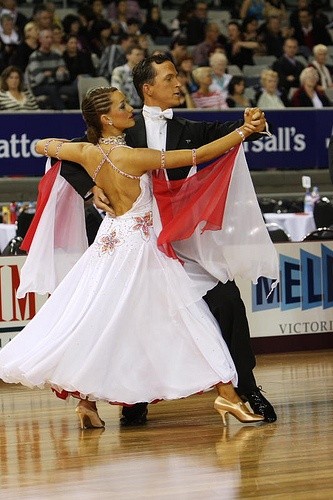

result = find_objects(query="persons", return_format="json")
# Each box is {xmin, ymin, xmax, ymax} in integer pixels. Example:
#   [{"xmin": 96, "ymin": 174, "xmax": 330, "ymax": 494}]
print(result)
[
  {"xmin": 303, "ymin": 44, "xmax": 333, "ymax": 88},
  {"xmin": 289, "ymin": 66, "xmax": 333, "ymax": 108},
  {"xmin": 252, "ymin": 68, "xmax": 289, "ymax": 109},
  {"xmin": 187, "ymin": 67, "xmax": 230, "ymax": 109},
  {"xmin": 0, "ymin": 0, "xmax": 333, "ymax": 78},
  {"xmin": 225, "ymin": 74, "xmax": 256, "ymax": 109},
  {"xmin": 0, "ymin": 65, "xmax": 43, "ymax": 112},
  {"xmin": 33, "ymin": 87, "xmax": 268, "ymax": 430},
  {"xmin": 56, "ymin": 51, "xmax": 278, "ymax": 427},
  {"xmin": 110, "ymin": 46, "xmax": 147, "ymax": 109},
  {"xmin": 167, "ymin": 72, "xmax": 195, "ymax": 109},
  {"xmin": 269, "ymin": 39, "xmax": 306, "ymax": 95},
  {"xmin": 24, "ymin": 29, "xmax": 79, "ymax": 110},
  {"xmin": 195, "ymin": 53, "xmax": 235, "ymax": 96}
]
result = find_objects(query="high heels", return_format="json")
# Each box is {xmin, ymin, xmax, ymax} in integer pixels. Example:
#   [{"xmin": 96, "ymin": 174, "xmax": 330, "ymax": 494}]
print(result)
[
  {"xmin": 78, "ymin": 402, "xmax": 105, "ymax": 429},
  {"xmin": 213, "ymin": 397, "xmax": 264, "ymax": 426}
]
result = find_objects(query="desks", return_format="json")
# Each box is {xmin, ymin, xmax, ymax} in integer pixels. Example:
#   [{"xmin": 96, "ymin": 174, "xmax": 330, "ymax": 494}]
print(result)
[
  {"xmin": 0, "ymin": 223, "xmax": 19, "ymax": 254},
  {"xmin": 263, "ymin": 213, "xmax": 316, "ymax": 242}
]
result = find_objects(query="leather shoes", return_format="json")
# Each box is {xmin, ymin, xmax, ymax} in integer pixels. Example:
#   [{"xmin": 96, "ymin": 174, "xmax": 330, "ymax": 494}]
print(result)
[
  {"xmin": 119, "ymin": 404, "xmax": 149, "ymax": 424},
  {"xmin": 239, "ymin": 385, "xmax": 277, "ymax": 422}
]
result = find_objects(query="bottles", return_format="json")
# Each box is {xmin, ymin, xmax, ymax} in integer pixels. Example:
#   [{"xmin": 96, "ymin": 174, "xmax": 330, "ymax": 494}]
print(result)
[
  {"xmin": 9, "ymin": 199, "xmax": 35, "ymax": 223},
  {"xmin": 304, "ymin": 184, "xmax": 320, "ymax": 213}
]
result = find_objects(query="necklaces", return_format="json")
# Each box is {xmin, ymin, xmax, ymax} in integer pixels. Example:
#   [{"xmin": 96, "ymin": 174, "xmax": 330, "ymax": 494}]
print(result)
[{"xmin": 97, "ymin": 131, "xmax": 129, "ymax": 144}]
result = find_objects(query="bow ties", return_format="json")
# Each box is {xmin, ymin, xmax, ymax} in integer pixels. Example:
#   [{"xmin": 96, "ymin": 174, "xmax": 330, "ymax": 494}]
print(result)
[{"xmin": 142, "ymin": 105, "xmax": 175, "ymax": 119}]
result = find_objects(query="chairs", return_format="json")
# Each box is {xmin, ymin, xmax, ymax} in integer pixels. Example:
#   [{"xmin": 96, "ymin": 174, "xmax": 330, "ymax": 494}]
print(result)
[
  {"xmin": 9, "ymin": 207, "xmax": 36, "ymax": 256},
  {"xmin": 258, "ymin": 197, "xmax": 333, "ymax": 242},
  {"xmin": 13, "ymin": 8, "xmax": 333, "ymax": 109}
]
